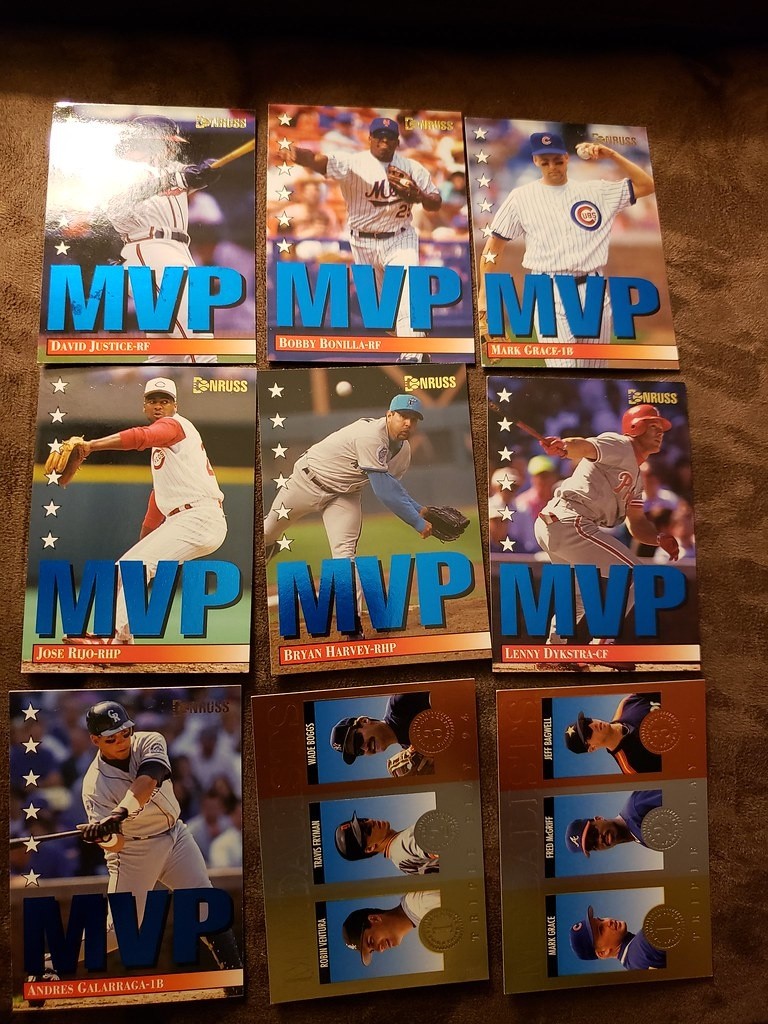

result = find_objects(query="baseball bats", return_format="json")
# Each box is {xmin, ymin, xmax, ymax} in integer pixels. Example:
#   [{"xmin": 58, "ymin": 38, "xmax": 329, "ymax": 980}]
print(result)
[
  {"xmin": 487, "ymin": 397, "xmax": 568, "ymax": 459},
  {"xmin": 9, "ymin": 822, "xmax": 123, "ymax": 851},
  {"xmin": 211, "ymin": 138, "xmax": 256, "ymax": 169}
]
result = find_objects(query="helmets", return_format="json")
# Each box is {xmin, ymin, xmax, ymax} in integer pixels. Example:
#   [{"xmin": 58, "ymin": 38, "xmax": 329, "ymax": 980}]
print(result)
[
  {"xmin": 622, "ymin": 405, "xmax": 672, "ymax": 437},
  {"xmin": 86, "ymin": 702, "xmax": 137, "ymax": 737},
  {"xmin": 127, "ymin": 116, "xmax": 189, "ymax": 144}
]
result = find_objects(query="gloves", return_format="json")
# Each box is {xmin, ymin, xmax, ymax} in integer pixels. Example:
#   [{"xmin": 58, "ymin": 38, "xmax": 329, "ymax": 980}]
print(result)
[
  {"xmin": 75, "ymin": 807, "xmax": 131, "ymax": 842},
  {"xmin": 176, "ymin": 158, "xmax": 223, "ymax": 191}
]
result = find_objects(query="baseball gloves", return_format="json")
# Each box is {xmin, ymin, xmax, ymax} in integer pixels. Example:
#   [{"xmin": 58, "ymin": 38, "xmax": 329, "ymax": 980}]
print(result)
[
  {"xmin": 45, "ymin": 433, "xmax": 87, "ymax": 485},
  {"xmin": 424, "ymin": 504, "xmax": 471, "ymax": 545},
  {"xmin": 386, "ymin": 747, "xmax": 434, "ymax": 777},
  {"xmin": 388, "ymin": 165, "xmax": 422, "ymax": 205}
]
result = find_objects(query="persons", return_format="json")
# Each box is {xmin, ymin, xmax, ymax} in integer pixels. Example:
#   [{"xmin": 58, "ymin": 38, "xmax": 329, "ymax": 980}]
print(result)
[
  {"xmin": 100, "ymin": 114, "xmax": 658, "ymax": 367},
  {"xmin": 10, "ymin": 379, "xmax": 694, "ymax": 983}
]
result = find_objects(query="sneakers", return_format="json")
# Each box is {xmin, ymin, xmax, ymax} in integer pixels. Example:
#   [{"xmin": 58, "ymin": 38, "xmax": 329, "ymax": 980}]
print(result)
[{"xmin": 61, "ymin": 633, "xmax": 106, "ymax": 645}]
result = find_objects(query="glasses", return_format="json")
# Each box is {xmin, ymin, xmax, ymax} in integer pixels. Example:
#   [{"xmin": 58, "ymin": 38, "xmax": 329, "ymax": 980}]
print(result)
[
  {"xmin": 582, "ymin": 716, "xmax": 592, "ymax": 741},
  {"xmin": 373, "ymin": 130, "xmax": 394, "ymax": 138},
  {"xmin": 352, "ymin": 730, "xmax": 365, "ymax": 759}
]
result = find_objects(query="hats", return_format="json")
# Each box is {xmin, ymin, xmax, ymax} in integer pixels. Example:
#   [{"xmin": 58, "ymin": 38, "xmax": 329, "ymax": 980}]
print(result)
[
  {"xmin": 335, "ymin": 811, "xmax": 363, "ymax": 860},
  {"xmin": 565, "ymin": 711, "xmax": 585, "ymax": 755},
  {"xmin": 329, "ymin": 716, "xmax": 357, "ymax": 766},
  {"xmin": 370, "ymin": 118, "xmax": 398, "ymax": 138},
  {"xmin": 143, "ymin": 377, "xmax": 177, "ymax": 401},
  {"xmin": 570, "ymin": 906, "xmax": 596, "ymax": 961},
  {"xmin": 342, "ymin": 908, "xmax": 373, "ymax": 967},
  {"xmin": 530, "ymin": 132, "xmax": 566, "ymax": 154},
  {"xmin": 565, "ymin": 819, "xmax": 591, "ymax": 858},
  {"xmin": 389, "ymin": 394, "xmax": 425, "ymax": 422}
]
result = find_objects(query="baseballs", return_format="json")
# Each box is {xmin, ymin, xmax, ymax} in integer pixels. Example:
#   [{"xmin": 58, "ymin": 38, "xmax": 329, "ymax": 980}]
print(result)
[{"xmin": 576, "ymin": 147, "xmax": 591, "ymax": 160}]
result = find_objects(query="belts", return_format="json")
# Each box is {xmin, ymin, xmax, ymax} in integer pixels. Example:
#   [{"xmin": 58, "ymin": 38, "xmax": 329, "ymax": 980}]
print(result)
[
  {"xmin": 155, "ymin": 230, "xmax": 188, "ymax": 242},
  {"xmin": 575, "ymin": 272, "xmax": 598, "ymax": 285},
  {"xmin": 304, "ymin": 468, "xmax": 334, "ymax": 493},
  {"xmin": 351, "ymin": 227, "xmax": 406, "ymax": 239},
  {"xmin": 169, "ymin": 504, "xmax": 193, "ymax": 516},
  {"xmin": 134, "ymin": 829, "xmax": 170, "ymax": 840}
]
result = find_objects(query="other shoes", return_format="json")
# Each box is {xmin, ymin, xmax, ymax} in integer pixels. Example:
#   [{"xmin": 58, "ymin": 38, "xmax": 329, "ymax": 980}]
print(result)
[
  {"xmin": 24, "ymin": 954, "xmax": 56, "ymax": 1007},
  {"xmin": 347, "ymin": 616, "xmax": 366, "ymax": 641}
]
